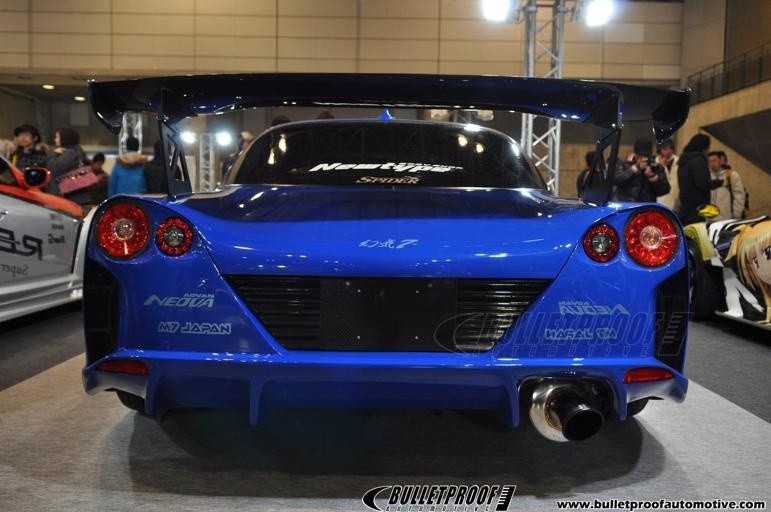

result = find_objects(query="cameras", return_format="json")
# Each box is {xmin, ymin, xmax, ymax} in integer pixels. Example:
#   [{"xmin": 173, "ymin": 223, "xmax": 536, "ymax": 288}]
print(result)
[{"xmin": 646, "ymin": 156, "xmax": 660, "ymax": 173}]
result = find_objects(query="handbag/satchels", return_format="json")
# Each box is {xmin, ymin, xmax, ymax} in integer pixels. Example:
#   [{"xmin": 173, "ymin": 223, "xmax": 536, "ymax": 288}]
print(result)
[{"xmin": 57, "ymin": 165, "xmax": 98, "ymax": 194}]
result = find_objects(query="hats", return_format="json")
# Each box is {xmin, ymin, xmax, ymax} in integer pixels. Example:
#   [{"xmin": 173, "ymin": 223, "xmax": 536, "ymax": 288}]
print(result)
[
  {"xmin": 14, "ymin": 124, "xmax": 43, "ymax": 145},
  {"xmin": 634, "ymin": 139, "xmax": 652, "ymax": 155}
]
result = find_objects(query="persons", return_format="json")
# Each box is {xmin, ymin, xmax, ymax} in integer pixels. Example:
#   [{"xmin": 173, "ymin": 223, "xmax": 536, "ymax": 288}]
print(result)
[
  {"xmin": 0, "ymin": 124, "xmax": 183, "ymax": 217},
  {"xmin": 576, "ymin": 131, "xmax": 750, "ymax": 227},
  {"xmin": 317, "ymin": 110, "xmax": 336, "ymax": 120},
  {"xmin": 220, "ymin": 131, "xmax": 255, "ymax": 184},
  {"xmin": 270, "ymin": 114, "xmax": 291, "ymax": 125}
]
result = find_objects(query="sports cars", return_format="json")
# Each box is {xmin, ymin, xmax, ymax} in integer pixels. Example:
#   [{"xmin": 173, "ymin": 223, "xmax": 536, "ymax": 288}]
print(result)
[
  {"xmin": 683, "ymin": 204, "xmax": 771, "ymax": 329},
  {"xmin": 0, "ymin": 71, "xmax": 698, "ymax": 443}
]
